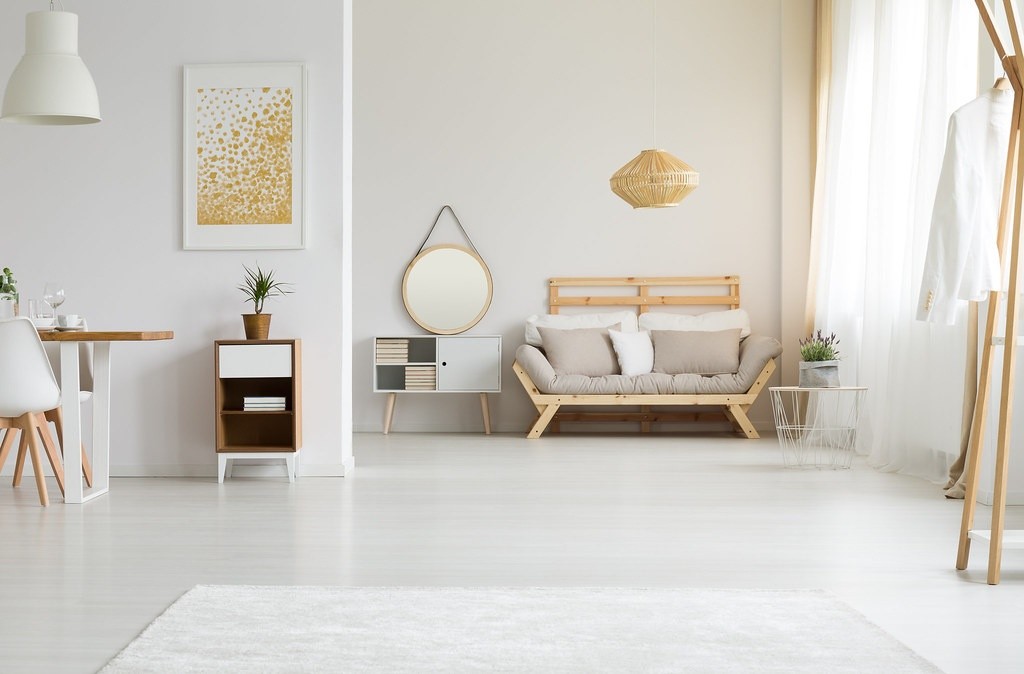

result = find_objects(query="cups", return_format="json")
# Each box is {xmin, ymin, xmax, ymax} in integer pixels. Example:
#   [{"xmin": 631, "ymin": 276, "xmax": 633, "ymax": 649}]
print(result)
[
  {"xmin": 58, "ymin": 314, "xmax": 79, "ymax": 326},
  {"xmin": 29, "ymin": 300, "xmax": 42, "ymax": 319}
]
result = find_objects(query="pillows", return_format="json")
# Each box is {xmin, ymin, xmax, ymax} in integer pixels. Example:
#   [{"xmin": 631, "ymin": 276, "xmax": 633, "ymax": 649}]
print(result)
[
  {"xmin": 536, "ymin": 323, "xmax": 621, "ymax": 378},
  {"xmin": 609, "ymin": 329, "xmax": 654, "ymax": 376},
  {"xmin": 651, "ymin": 329, "xmax": 742, "ymax": 375}
]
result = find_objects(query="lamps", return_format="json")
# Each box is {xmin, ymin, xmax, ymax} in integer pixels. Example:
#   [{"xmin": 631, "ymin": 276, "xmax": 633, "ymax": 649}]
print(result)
[
  {"xmin": 610, "ymin": 0, "xmax": 699, "ymax": 209},
  {"xmin": 0, "ymin": 0, "xmax": 102, "ymax": 127}
]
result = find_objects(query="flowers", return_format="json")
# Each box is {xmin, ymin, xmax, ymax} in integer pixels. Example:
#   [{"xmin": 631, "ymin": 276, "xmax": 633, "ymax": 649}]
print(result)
[{"xmin": 799, "ymin": 329, "xmax": 841, "ymax": 362}]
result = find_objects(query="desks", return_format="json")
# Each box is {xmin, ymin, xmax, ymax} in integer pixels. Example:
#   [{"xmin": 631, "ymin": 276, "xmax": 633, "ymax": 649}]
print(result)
[
  {"xmin": 37, "ymin": 330, "xmax": 175, "ymax": 504},
  {"xmin": 769, "ymin": 386, "xmax": 869, "ymax": 472}
]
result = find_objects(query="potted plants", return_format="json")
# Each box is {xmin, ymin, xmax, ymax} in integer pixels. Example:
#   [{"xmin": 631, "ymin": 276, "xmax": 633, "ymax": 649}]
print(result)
[
  {"xmin": 0, "ymin": 266, "xmax": 17, "ymax": 318},
  {"xmin": 236, "ymin": 261, "xmax": 297, "ymax": 340}
]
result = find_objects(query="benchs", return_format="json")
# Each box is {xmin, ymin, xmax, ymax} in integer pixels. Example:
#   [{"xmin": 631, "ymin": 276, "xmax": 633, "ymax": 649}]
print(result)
[{"xmin": 511, "ymin": 277, "xmax": 783, "ymax": 439}]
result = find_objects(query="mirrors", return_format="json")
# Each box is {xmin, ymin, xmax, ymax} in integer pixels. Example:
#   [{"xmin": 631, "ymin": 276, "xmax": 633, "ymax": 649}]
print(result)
[{"xmin": 401, "ymin": 206, "xmax": 493, "ymax": 335}]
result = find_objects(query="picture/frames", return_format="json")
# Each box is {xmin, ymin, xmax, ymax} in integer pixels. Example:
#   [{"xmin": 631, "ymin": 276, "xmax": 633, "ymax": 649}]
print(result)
[{"xmin": 182, "ymin": 62, "xmax": 305, "ymax": 251}]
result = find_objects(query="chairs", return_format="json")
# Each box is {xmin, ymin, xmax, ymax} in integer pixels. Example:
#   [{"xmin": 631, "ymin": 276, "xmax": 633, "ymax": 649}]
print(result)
[{"xmin": 1, "ymin": 314, "xmax": 92, "ymax": 505}]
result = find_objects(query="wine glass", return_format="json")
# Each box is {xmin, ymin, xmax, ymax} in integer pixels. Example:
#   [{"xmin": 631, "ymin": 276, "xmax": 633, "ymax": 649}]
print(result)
[{"xmin": 44, "ymin": 287, "xmax": 65, "ymax": 332}]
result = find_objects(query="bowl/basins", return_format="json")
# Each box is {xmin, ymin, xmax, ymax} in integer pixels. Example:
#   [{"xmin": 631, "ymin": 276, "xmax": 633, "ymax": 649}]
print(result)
[{"xmin": 30, "ymin": 317, "xmax": 56, "ymax": 326}]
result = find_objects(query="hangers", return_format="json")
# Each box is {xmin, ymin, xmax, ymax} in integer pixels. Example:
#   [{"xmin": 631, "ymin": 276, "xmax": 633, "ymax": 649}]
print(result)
[{"xmin": 993, "ymin": 54, "xmax": 1012, "ymax": 89}]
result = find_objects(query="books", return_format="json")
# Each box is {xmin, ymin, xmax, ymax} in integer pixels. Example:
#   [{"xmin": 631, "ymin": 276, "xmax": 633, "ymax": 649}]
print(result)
[
  {"xmin": 376, "ymin": 339, "xmax": 409, "ymax": 363},
  {"xmin": 405, "ymin": 366, "xmax": 436, "ymax": 390},
  {"xmin": 243, "ymin": 396, "xmax": 286, "ymax": 412}
]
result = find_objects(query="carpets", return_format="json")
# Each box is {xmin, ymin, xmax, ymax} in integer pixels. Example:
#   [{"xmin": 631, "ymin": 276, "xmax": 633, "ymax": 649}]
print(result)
[{"xmin": 93, "ymin": 582, "xmax": 943, "ymax": 674}]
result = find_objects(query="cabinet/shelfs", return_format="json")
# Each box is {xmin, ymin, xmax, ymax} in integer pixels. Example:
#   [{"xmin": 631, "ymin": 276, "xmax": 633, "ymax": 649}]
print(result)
[
  {"xmin": 372, "ymin": 335, "xmax": 503, "ymax": 435},
  {"xmin": 214, "ymin": 339, "xmax": 302, "ymax": 483}
]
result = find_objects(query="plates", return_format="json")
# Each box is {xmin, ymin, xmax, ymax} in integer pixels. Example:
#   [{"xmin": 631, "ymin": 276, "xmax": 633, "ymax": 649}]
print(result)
[
  {"xmin": 55, "ymin": 326, "xmax": 84, "ymax": 332},
  {"xmin": 36, "ymin": 326, "xmax": 56, "ymax": 333}
]
